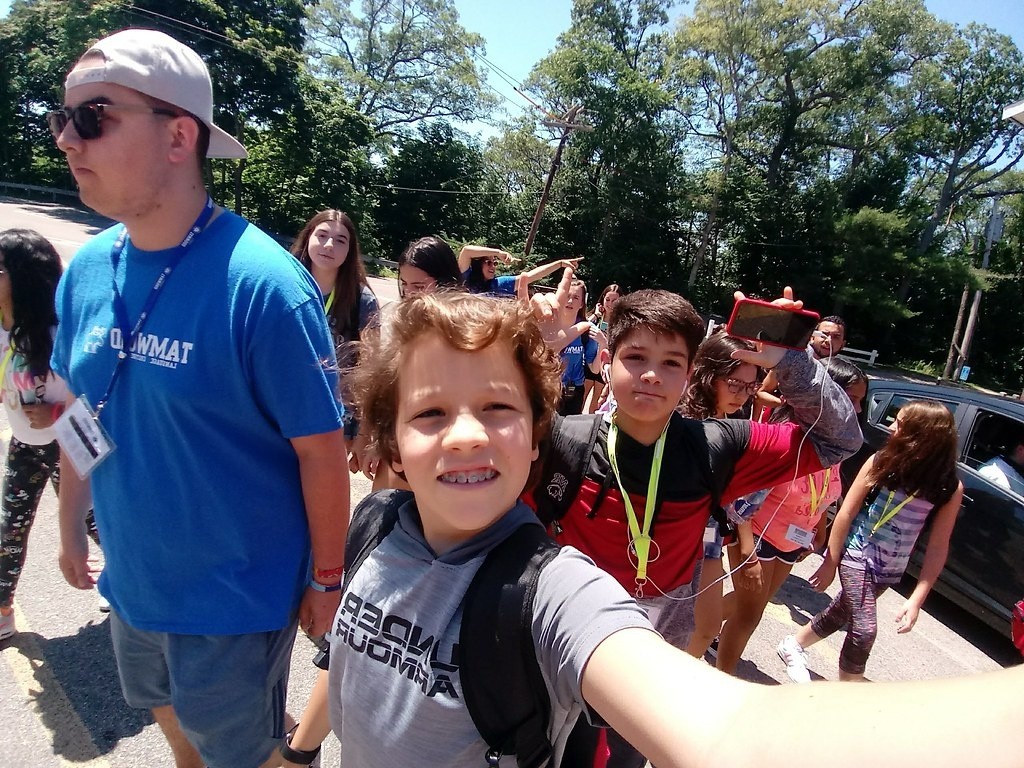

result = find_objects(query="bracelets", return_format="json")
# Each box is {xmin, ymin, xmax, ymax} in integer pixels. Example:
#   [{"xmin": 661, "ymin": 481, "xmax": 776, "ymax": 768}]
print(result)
[
  {"xmin": 313, "ymin": 564, "xmax": 345, "ymax": 578},
  {"xmin": 561, "ymin": 259, "xmax": 562, "ymax": 269},
  {"xmin": 278, "ymin": 723, "xmax": 321, "ymax": 765},
  {"xmin": 308, "ymin": 578, "xmax": 342, "ymax": 592},
  {"xmin": 594, "ymin": 313, "xmax": 599, "ymax": 320},
  {"xmin": 740, "ymin": 556, "xmax": 759, "ymax": 564}
]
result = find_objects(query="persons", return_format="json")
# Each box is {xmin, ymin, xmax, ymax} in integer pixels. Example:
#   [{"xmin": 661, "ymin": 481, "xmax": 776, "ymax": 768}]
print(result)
[
  {"xmin": 50, "ymin": 27, "xmax": 352, "ymax": 768},
  {"xmin": 776, "ymin": 400, "xmax": 963, "ymax": 685},
  {"xmin": 289, "ymin": 209, "xmax": 872, "ymax": 768},
  {"xmin": 976, "ymin": 431, "xmax": 1024, "ymax": 498},
  {"xmin": 0, "ymin": 229, "xmax": 111, "ymax": 642},
  {"xmin": 281, "ymin": 285, "xmax": 1024, "ymax": 767}
]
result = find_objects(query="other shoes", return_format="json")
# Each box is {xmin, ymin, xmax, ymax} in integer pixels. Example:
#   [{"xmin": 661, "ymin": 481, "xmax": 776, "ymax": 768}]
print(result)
[
  {"xmin": 0, "ymin": 608, "xmax": 15, "ymax": 641},
  {"xmin": 98, "ymin": 596, "xmax": 112, "ymax": 611},
  {"xmin": 707, "ymin": 634, "xmax": 719, "ymax": 658}
]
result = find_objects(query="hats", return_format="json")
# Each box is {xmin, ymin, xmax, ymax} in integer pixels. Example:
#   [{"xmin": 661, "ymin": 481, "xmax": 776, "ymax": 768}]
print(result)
[{"xmin": 67, "ymin": 27, "xmax": 250, "ymax": 159}]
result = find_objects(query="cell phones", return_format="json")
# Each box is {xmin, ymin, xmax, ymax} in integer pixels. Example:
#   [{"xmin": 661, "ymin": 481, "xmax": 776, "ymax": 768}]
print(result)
[{"xmin": 727, "ymin": 298, "xmax": 819, "ymax": 352}]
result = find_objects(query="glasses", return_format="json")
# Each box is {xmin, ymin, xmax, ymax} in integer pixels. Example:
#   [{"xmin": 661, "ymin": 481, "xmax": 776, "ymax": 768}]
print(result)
[
  {"xmin": 45, "ymin": 99, "xmax": 179, "ymax": 145},
  {"xmin": 721, "ymin": 376, "xmax": 762, "ymax": 396},
  {"xmin": 484, "ymin": 260, "xmax": 499, "ymax": 267}
]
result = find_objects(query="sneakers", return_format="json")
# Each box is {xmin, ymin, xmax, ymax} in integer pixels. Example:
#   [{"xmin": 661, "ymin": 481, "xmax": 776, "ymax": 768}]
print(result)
[{"xmin": 776, "ymin": 637, "xmax": 812, "ymax": 684}]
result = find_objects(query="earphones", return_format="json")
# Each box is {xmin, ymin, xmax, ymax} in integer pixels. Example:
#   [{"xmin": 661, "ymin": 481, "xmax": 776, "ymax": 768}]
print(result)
[
  {"xmin": 603, "ymin": 363, "xmax": 610, "ymax": 382},
  {"xmin": 681, "ymin": 380, "xmax": 688, "ymax": 397}
]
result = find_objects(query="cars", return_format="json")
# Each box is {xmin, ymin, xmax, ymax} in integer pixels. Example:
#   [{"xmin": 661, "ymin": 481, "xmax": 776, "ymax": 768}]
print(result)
[{"xmin": 818, "ymin": 375, "xmax": 1023, "ymax": 641}]
